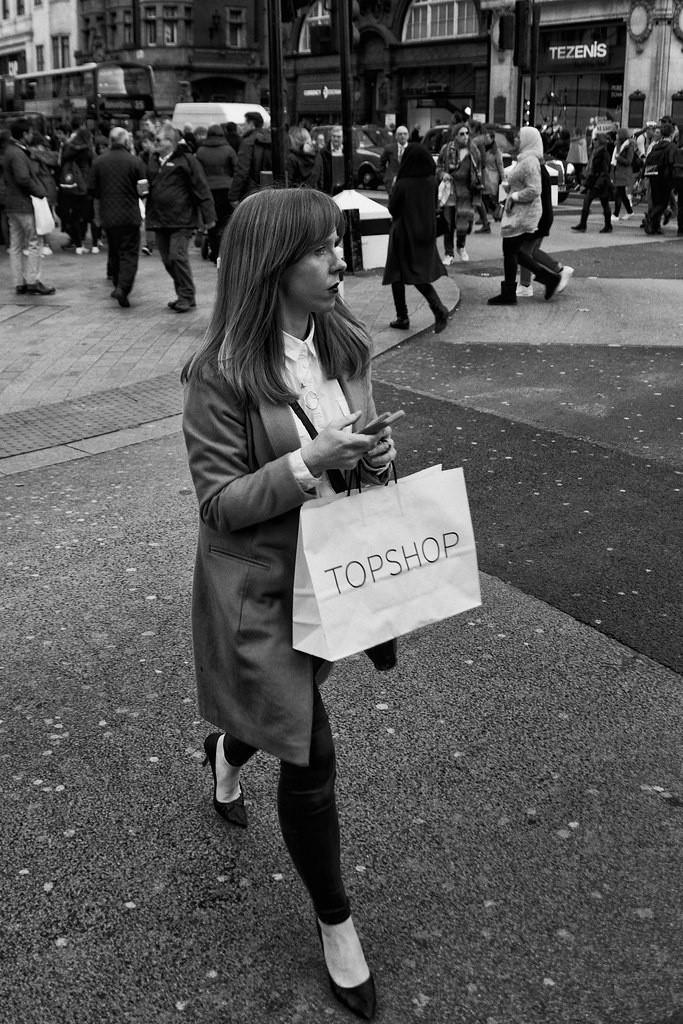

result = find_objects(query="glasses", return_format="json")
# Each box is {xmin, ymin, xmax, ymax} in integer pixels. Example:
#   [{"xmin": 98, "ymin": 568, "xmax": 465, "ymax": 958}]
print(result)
[
  {"xmin": 153, "ymin": 138, "xmax": 168, "ymax": 143},
  {"xmin": 594, "ymin": 138, "xmax": 598, "ymax": 141},
  {"xmin": 457, "ymin": 132, "xmax": 469, "ymax": 136}
]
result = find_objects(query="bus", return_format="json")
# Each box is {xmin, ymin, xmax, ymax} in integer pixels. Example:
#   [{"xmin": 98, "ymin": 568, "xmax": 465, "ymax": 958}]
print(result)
[{"xmin": 0, "ymin": 60, "xmax": 159, "ymax": 139}]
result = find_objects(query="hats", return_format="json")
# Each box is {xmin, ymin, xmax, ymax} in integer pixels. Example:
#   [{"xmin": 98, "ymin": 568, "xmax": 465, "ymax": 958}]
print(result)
[
  {"xmin": 452, "ymin": 123, "xmax": 470, "ymax": 139},
  {"xmin": 597, "ymin": 115, "xmax": 675, "ymax": 145}
]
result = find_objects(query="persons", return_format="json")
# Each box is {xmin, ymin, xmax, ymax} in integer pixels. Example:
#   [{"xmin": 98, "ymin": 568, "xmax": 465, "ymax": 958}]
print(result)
[
  {"xmin": 180, "ymin": 192, "xmax": 398, "ymax": 1023},
  {"xmin": 0, "ymin": 112, "xmax": 347, "ymax": 312},
  {"xmin": 379, "ymin": 111, "xmax": 683, "ymax": 334}
]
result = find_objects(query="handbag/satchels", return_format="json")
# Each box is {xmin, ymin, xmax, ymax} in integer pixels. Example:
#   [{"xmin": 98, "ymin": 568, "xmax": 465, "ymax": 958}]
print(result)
[
  {"xmin": 30, "ymin": 195, "xmax": 55, "ymax": 235},
  {"xmin": 292, "ymin": 438, "xmax": 482, "ymax": 662}
]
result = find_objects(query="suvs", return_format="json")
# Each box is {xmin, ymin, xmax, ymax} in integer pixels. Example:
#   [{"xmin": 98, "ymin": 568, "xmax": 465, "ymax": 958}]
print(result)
[{"xmin": 417, "ymin": 122, "xmax": 572, "ymax": 206}]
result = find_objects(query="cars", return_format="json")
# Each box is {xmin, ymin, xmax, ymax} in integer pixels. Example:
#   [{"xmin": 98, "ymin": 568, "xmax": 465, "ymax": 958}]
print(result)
[{"xmin": 310, "ymin": 124, "xmax": 389, "ymax": 191}]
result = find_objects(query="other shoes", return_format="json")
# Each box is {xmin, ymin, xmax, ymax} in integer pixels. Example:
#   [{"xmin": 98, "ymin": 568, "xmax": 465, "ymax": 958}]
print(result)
[
  {"xmin": 434, "ymin": 306, "xmax": 449, "ymax": 334},
  {"xmin": 168, "ymin": 297, "xmax": 196, "ymax": 311},
  {"xmin": 6, "ymin": 240, "xmax": 152, "ymax": 257},
  {"xmin": 111, "ymin": 289, "xmax": 115, "ymax": 298},
  {"xmin": 115, "ymin": 288, "xmax": 131, "ymax": 308},
  {"xmin": 27, "ymin": 279, "xmax": 56, "ymax": 296},
  {"xmin": 390, "ymin": 318, "xmax": 409, "ymax": 330},
  {"xmin": 16, "ymin": 284, "xmax": 27, "ymax": 294},
  {"xmin": 571, "ymin": 211, "xmax": 672, "ymax": 235},
  {"xmin": 475, "ymin": 204, "xmax": 504, "ymax": 233}
]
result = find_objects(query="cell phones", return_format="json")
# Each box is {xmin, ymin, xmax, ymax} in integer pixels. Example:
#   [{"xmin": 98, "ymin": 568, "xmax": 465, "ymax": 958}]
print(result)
[{"xmin": 356, "ymin": 410, "xmax": 406, "ymax": 436}]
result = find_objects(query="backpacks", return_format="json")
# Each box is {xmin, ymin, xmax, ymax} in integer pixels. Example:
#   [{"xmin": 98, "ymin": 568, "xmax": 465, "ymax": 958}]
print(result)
[
  {"xmin": 253, "ymin": 131, "xmax": 273, "ymax": 184},
  {"xmin": 631, "ymin": 151, "xmax": 643, "ymax": 173}
]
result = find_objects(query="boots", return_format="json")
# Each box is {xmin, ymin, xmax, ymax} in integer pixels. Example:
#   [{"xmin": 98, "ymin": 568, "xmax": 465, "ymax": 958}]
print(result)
[
  {"xmin": 487, "ymin": 281, "xmax": 518, "ymax": 306},
  {"xmin": 533, "ymin": 267, "xmax": 562, "ymax": 301}
]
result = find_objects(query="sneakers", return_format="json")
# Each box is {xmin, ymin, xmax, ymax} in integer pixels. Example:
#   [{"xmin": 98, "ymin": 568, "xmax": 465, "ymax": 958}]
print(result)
[
  {"xmin": 516, "ymin": 285, "xmax": 534, "ymax": 297},
  {"xmin": 441, "ymin": 255, "xmax": 455, "ymax": 265},
  {"xmin": 457, "ymin": 247, "xmax": 469, "ymax": 262},
  {"xmin": 557, "ymin": 266, "xmax": 574, "ymax": 293}
]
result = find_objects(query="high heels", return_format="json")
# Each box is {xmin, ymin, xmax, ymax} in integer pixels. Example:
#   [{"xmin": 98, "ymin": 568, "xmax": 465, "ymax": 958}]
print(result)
[
  {"xmin": 204, "ymin": 733, "xmax": 248, "ymax": 830},
  {"xmin": 315, "ymin": 916, "xmax": 376, "ymax": 1024}
]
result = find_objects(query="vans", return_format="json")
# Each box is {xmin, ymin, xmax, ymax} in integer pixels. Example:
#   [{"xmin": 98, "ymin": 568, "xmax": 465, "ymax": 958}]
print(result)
[{"xmin": 169, "ymin": 100, "xmax": 272, "ymax": 142}]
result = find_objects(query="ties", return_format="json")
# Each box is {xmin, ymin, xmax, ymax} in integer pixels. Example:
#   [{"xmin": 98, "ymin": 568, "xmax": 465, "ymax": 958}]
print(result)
[{"xmin": 399, "ymin": 147, "xmax": 403, "ymax": 161}]
